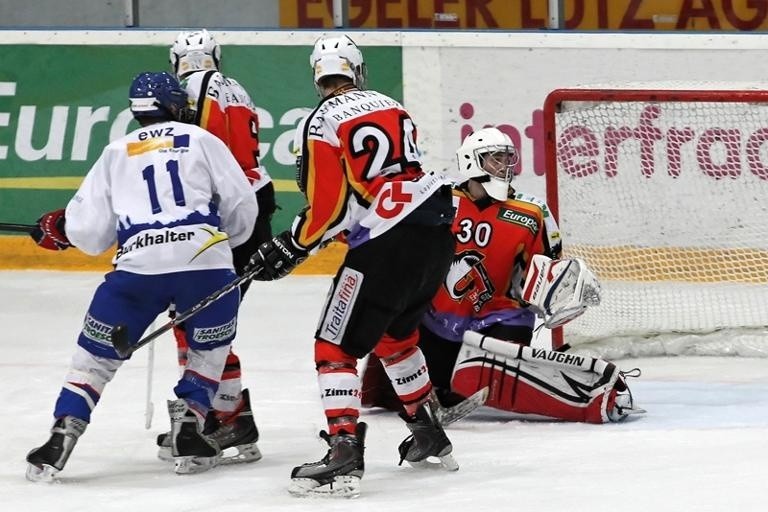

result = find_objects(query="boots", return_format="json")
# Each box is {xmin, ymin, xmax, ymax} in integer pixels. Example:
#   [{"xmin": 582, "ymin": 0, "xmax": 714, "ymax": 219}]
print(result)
[
  {"xmin": 288, "ymin": 420, "xmax": 371, "ymax": 482},
  {"xmin": 24, "ymin": 412, "xmax": 92, "ymax": 471},
  {"xmin": 161, "ymin": 397, "xmax": 228, "ymax": 457},
  {"xmin": 154, "ymin": 386, "xmax": 261, "ymax": 449},
  {"xmin": 392, "ymin": 399, "xmax": 455, "ymax": 462}
]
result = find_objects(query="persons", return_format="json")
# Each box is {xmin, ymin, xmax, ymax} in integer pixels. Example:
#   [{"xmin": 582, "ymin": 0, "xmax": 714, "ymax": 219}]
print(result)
[
  {"xmin": 20, "ymin": 69, "xmax": 259, "ymax": 474},
  {"xmin": 156, "ymin": 26, "xmax": 278, "ymax": 456},
  {"xmin": 238, "ymin": 30, "xmax": 453, "ymax": 487},
  {"xmin": 361, "ymin": 127, "xmax": 565, "ymax": 411}
]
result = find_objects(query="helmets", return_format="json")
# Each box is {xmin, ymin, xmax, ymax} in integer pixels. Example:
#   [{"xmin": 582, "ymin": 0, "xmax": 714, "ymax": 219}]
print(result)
[
  {"xmin": 454, "ymin": 125, "xmax": 519, "ymax": 186},
  {"xmin": 127, "ymin": 72, "xmax": 188, "ymax": 124},
  {"xmin": 168, "ymin": 29, "xmax": 222, "ymax": 78},
  {"xmin": 309, "ymin": 32, "xmax": 364, "ymax": 88}
]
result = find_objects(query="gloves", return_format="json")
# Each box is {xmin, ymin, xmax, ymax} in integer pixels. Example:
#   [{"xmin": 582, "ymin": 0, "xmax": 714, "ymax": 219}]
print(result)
[
  {"xmin": 240, "ymin": 230, "xmax": 311, "ymax": 283},
  {"xmin": 27, "ymin": 207, "xmax": 75, "ymax": 257}
]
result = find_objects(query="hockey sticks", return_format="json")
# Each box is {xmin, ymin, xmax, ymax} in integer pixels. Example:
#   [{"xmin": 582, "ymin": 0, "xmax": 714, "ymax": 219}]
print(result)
[
  {"xmin": 111, "ymin": 263, "xmax": 265, "ymax": 361},
  {"xmin": 146, "ymin": 319, "xmax": 154, "ymax": 423},
  {"xmin": 430, "ymin": 386, "xmax": 489, "ymax": 428}
]
